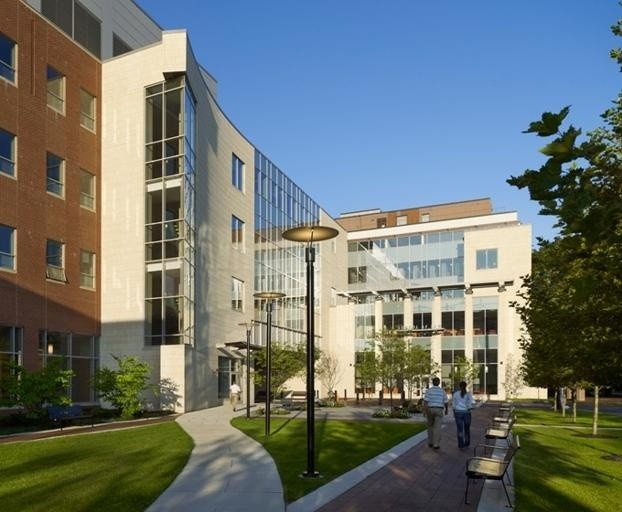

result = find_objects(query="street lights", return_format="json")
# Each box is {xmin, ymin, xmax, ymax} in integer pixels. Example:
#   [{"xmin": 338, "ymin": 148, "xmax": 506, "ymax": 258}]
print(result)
[
  {"xmin": 282, "ymin": 226, "xmax": 338, "ymax": 480},
  {"xmin": 238, "ymin": 323, "xmax": 260, "ymax": 420},
  {"xmin": 253, "ymin": 292, "xmax": 286, "ymax": 438}
]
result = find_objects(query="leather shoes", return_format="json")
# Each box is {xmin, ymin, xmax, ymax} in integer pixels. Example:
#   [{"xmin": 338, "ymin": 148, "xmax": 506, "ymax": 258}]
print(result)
[{"xmin": 428, "ymin": 443, "xmax": 440, "ymax": 450}]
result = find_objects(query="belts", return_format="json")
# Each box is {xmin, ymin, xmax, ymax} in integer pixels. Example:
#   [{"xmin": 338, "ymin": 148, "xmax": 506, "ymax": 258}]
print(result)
[{"xmin": 428, "ymin": 406, "xmax": 444, "ymax": 409}]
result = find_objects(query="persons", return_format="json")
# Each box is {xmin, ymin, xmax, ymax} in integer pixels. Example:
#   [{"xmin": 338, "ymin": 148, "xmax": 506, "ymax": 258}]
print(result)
[
  {"xmin": 451, "ymin": 381, "xmax": 474, "ymax": 451},
  {"xmin": 229, "ymin": 382, "xmax": 240, "ymax": 411},
  {"xmin": 423, "ymin": 377, "xmax": 449, "ymax": 449}
]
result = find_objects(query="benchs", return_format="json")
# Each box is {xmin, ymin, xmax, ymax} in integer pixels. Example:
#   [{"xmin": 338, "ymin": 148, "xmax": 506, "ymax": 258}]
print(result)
[
  {"xmin": 283, "ymin": 391, "xmax": 307, "ymax": 401},
  {"xmin": 59, "ymin": 414, "xmax": 93, "ymax": 431}
]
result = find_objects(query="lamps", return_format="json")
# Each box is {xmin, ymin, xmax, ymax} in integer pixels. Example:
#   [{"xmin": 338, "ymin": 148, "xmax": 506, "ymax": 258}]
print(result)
[
  {"xmin": 464, "ymin": 283, "xmax": 472, "ymax": 294},
  {"xmin": 432, "ymin": 285, "xmax": 441, "ymax": 296},
  {"xmin": 498, "ymin": 280, "xmax": 505, "ymax": 292}
]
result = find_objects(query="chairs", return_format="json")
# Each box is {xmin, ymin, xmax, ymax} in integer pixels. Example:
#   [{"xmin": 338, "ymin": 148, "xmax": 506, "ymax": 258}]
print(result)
[
  {"xmin": 464, "ymin": 434, "xmax": 520, "ymax": 508},
  {"xmin": 499, "ymin": 403, "xmax": 512, "ymax": 417},
  {"xmin": 483, "ymin": 415, "xmax": 517, "ymax": 456},
  {"xmin": 501, "ymin": 402, "xmax": 512, "ymax": 407},
  {"xmin": 492, "ymin": 407, "xmax": 515, "ymax": 428}
]
